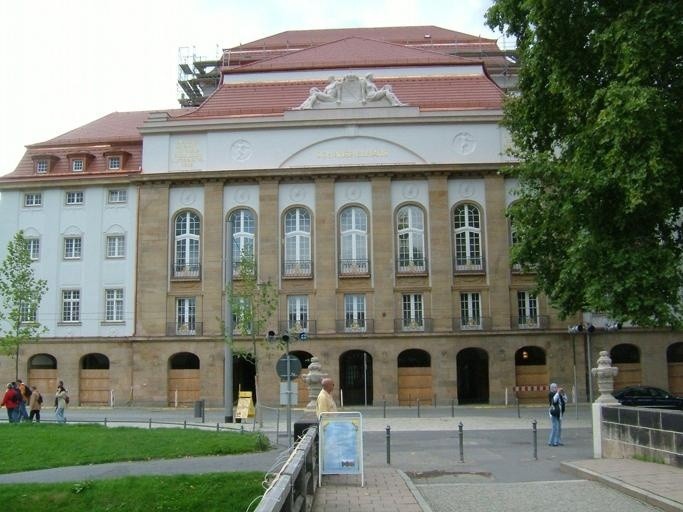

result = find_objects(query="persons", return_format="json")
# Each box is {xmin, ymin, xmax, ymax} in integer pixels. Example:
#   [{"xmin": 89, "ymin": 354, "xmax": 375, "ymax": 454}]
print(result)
[
  {"xmin": 55, "ymin": 380, "xmax": 64, "ymax": 415},
  {"xmin": 12, "ymin": 382, "xmax": 21, "ymax": 423},
  {"xmin": 315, "ymin": 377, "xmax": 338, "ymax": 419},
  {"xmin": 0, "ymin": 383, "xmax": 18, "ymax": 423},
  {"xmin": 291, "ymin": 75, "xmax": 341, "ymax": 111},
  {"xmin": 360, "ymin": 73, "xmax": 409, "ymax": 107},
  {"xmin": 17, "ymin": 380, "xmax": 29, "ymax": 420},
  {"xmin": 54, "ymin": 386, "xmax": 66, "ymax": 424},
  {"xmin": 28, "ymin": 385, "xmax": 43, "ymax": 423},
  {"xmin": 547, "ymin": 382, "xmax": 568, "ymax": 447}
]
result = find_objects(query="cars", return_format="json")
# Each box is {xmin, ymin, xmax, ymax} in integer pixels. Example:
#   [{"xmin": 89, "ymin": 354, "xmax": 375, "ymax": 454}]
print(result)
[{"xmin": 611, "ymin": 386, "xmax": 683, "ymax": 409}]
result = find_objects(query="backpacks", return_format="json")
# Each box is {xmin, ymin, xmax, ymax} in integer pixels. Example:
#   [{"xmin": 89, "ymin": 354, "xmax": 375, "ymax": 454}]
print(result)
[
  {"xmin": 21, "ymin": 384, "xmax": 32, "ymax": 397},
  {"xmin": 35, "ymin": 391, "xmax": 43, "ymax": 404}
]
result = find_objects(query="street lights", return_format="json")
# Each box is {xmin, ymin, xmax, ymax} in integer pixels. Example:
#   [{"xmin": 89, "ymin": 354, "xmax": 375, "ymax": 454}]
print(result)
[
  {"xmin": 266, "ymin": 331, "xmax": 309, "ymax": 447},
  {"xmin": 567, "ymin": 322, "xmax": 622, "ymax": 404}
]
